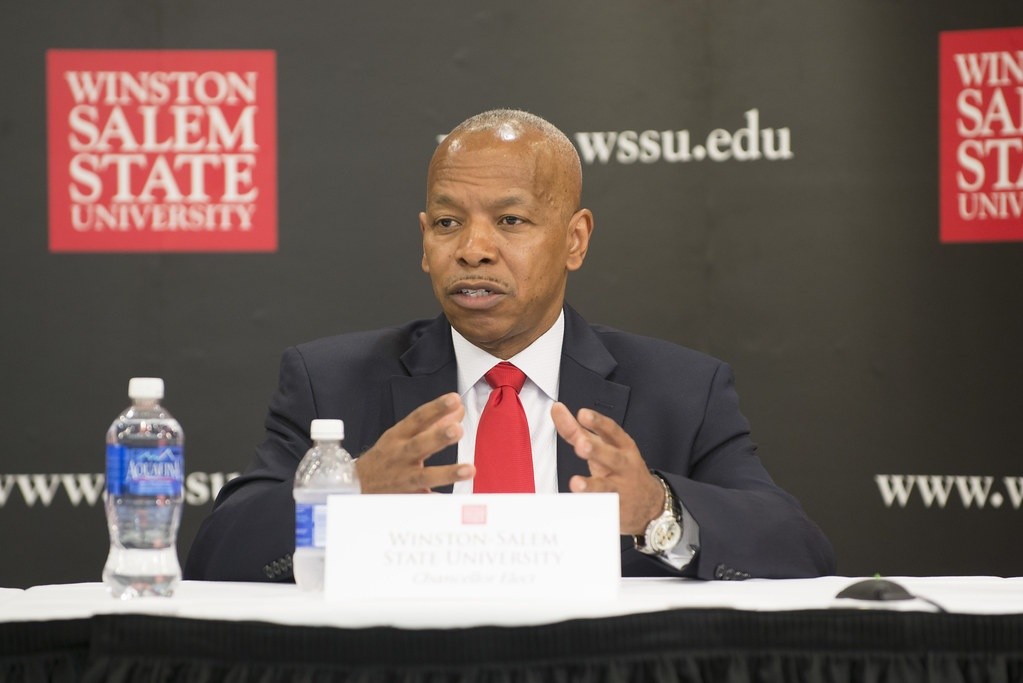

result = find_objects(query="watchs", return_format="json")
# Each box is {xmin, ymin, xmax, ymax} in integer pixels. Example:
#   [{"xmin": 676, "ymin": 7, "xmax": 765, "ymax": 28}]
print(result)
[{"xmin": 631, "ymin": 473, "xmax": 684, "ymax": 557}]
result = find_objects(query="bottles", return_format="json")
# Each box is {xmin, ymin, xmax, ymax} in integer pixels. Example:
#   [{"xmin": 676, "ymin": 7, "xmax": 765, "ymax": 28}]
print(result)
[
  {"xmin": 101, "ymin": 377, "xmax": 186, "ymax": 600},
  {"xmin": 291, "ymin": 419, "xmax": 359, "ymax": 592}
]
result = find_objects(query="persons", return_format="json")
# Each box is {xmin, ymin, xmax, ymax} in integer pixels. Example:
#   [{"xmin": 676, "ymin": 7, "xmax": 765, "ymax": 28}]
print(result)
[{"xmin": 184, "ymin": 107, "xmax": 835, "ymax": 586}]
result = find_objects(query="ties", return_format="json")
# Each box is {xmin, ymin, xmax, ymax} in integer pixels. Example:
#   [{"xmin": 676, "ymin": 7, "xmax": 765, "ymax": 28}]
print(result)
[{"xmin": 472, "ymin": 362, "xmax": 536, "ymax": 494}]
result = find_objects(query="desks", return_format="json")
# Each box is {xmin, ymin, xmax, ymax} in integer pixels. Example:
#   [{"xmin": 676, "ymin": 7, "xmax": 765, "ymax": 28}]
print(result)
[{"xmin": 0, "ymin": 574, "xmax": 1023, "ymax": 683}]
